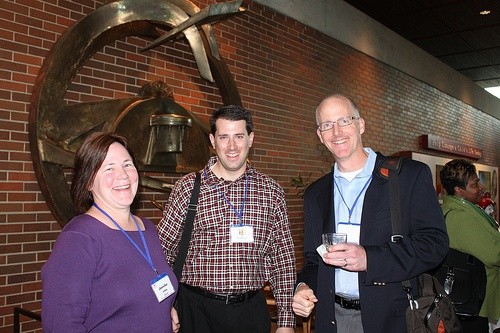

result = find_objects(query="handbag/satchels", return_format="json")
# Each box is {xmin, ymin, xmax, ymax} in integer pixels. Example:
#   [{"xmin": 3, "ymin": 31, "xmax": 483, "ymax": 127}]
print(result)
[
  {"xmin": 406, "ymin": 273, "xmax": 456, "ymax": 333},
  {"xmin": 428, "ymin": 246, "xmax": 487, "ymax": 318}
]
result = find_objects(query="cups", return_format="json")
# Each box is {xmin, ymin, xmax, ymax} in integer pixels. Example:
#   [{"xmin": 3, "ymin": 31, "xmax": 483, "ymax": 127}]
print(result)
[{"xmin": 322, "ymin": 233, "xmax": 347, "ymax": 252}]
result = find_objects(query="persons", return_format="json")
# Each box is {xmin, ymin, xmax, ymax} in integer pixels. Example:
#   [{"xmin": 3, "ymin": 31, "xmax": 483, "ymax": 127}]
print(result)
[
  {"xmin": 41, "ymin": 133, "xmax": 180, "ymax": 333},
  {"xmin": 157, "ymin": 104, "xmax": 297, "ymax": 333},
  {"xmin": 439, "ymin": 159, "xmax": 500, "ymax": 333},
  {"xmin": 291, "ymin": 94, "xmax": 449, "ymax": 333}
]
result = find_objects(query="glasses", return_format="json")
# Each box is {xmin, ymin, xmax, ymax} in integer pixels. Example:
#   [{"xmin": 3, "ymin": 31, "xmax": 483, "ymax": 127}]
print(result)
[{"xmin": 317, "ymin": 116, "xmax": 360, "ymax": 131}]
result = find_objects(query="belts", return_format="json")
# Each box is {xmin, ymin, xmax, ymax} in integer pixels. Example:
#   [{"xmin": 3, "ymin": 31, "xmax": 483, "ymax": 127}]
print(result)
[
  {"xmin": 180, "ymin": 282, "xmax": 262, "ymax": 303},
  {"xmin": 334, "ymin": 295, "xmax": 361, "ymax": 310}
]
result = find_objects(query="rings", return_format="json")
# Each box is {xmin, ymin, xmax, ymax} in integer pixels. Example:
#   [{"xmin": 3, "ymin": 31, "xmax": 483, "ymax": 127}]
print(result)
[{"xmin": 344, "ymin": 259, "xmax": 347, "ymax": 266}]
